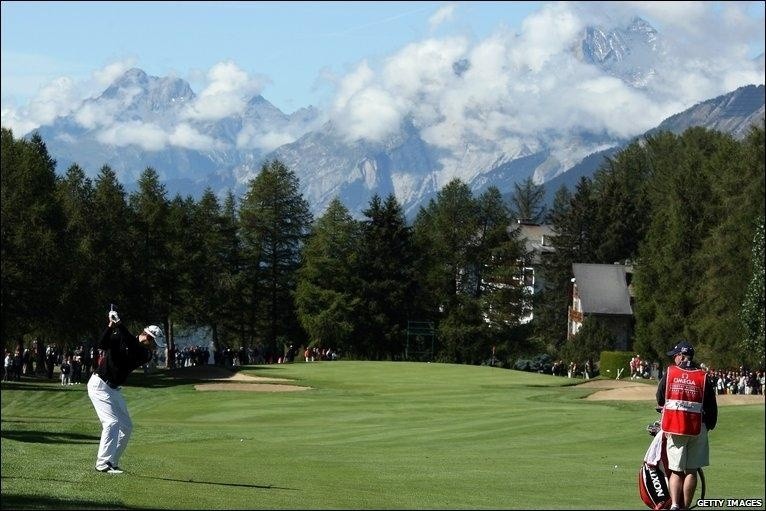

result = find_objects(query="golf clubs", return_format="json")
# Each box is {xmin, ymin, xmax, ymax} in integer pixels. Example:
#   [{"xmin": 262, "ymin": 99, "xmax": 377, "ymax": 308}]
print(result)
[
  {"xmin": 110, "ymin": 303, "xmax": 119, "ymax": 320},
  {"xmin": 646, "ymin": 420, "xmax": 661, "ymax": 437}
]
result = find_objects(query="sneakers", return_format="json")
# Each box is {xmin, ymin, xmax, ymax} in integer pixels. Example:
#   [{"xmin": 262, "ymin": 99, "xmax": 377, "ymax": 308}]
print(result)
[{"xmin": 95, "ymin": 463, "xmax": 124, "ymax": 474}]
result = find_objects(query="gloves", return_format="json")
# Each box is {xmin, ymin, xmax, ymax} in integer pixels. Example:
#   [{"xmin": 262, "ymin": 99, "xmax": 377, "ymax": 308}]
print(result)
[{"xmin": 109, "ymin": 310, "xmax": 120, "ymax": 323}]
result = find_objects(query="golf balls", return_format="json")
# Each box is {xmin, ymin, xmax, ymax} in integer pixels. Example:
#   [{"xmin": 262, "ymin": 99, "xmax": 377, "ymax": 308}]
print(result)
[{"xmin": 240, "ymin": 439, "xmax": 242, "ymax": 441}]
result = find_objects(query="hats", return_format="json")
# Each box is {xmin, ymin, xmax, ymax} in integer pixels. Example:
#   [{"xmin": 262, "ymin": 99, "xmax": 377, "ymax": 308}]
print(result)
[
  {"xmin": 144, "ymin": 325, "xmax": 166, "ymax": 349},
  {"xmin": 665, "ymin": 342, "xmax": 694, "ymax": 356}
]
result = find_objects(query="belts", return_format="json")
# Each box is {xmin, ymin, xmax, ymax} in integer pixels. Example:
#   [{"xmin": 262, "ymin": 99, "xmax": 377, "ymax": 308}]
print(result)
[{"xmin": 106, "ymin": 379, "xmax": 117, "ymax": 389}]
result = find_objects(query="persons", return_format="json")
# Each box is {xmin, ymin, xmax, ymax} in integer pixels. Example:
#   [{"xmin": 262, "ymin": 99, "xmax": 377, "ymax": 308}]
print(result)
[
  {"xmin": 0, "ymin": 338, "xmax": 337, "ymax": 387},
  {"xmin": 629, "ymin": 354, "xmax": 766, "ymax": 395},
  {"xmin": 656, "ymin": 340, "xmax": 719, "ymax": 510},
  {"xmin": 537, "ymin": 360, "xmax": 591, "ymax": 379},
  {"xmin": 86, "ymin": 309, "xmax": 166, "ymax": 474}
]
